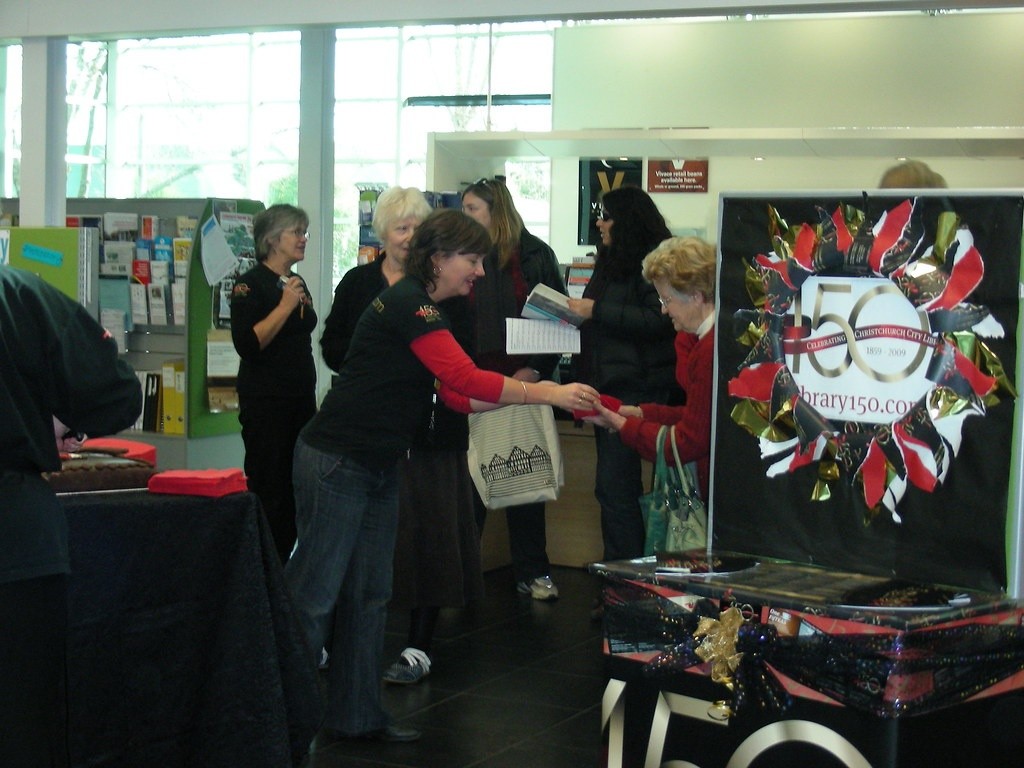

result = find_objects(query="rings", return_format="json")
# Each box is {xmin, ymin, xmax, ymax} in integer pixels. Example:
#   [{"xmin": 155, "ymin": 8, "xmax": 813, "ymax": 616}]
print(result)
[
  {"xmin": 295, "ymin": 279, "xmax": 300, "ymax": 284},
  {"xmin": 581, "ymin": 392, "xmax": 586, "ymax": 399},
  {"xmin": 578, "ymin": 398, "xmax": 582, "ymax": 403}
]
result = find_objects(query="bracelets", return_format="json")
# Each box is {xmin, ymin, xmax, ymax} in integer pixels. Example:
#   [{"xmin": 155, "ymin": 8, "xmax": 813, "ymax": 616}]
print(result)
[
  {"xmin": 520, "ymin": 380, "xmax": 528, "ymax": 404},
  {"xmin": 637, "ymin": 406, "xmax": 642, "ymax": 419}
]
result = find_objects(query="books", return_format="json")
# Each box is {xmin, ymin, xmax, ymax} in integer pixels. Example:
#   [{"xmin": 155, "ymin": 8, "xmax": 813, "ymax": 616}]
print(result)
[
  {"xmin": 519, "ymin": 283, "xmax": 584, "ymax": 329},
  {"xmin": 65, "ymin": 213, "xmax": 198, "ymax": 434}
]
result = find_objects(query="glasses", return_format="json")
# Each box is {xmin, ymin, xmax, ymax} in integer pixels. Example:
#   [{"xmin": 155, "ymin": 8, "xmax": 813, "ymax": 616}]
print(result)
[
  {"xmin": 599, "ymin": 210, "xmax": 610, "ymax": 221},
  {"xmin": 284, "ymin": 230, "xmax": 310, "ymax": 238},
  {"xmin": 477, "ymin": 177, "xmax": 493, "ymax": 190},
  {"xmin": 659, "ymin": 295, "xmax": 673, "ymax": 307}
]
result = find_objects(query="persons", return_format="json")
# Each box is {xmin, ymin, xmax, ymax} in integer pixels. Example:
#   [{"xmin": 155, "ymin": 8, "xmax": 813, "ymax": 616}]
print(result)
[
  {"xmin": 568, "ymin": 188, "xmax": 676, "ymax": 623},
  {"xmin": 0, "ymin": 263, "xmax": 143, "ymax": 768},
  {"xmin": 319, "ymin": 186, "xmax": 451, "ymax": 686},
  {"xmin": 581, "ymin": 237, "xmax": 716, "ymax": 507},
  {"xmin": 879, "ymin": 160, "xmax": 948, "ymax": 189},
  {"xmin": 278, "ymin": 208, "xmax": 602, "ymax": 768},
  {"xmin": 230, "ymin": 203, "xmax": 316, "ymax": 566},
  {"xmin": 462, "ymin": 179, "xmax": 569, "ymax": 617}
]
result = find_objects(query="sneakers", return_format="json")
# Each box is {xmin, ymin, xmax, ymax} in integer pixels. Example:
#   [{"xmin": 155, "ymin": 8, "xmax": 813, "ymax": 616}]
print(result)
[{"xmin": 515, "ymin": 575, "xmax": 558, "ymax": 599}]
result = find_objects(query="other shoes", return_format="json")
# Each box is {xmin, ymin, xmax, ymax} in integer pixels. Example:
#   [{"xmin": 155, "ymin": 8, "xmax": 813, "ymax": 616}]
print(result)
[
  {"xmin": 325, "ymin": 714, "xmax": 423, "ymax": 746},
  {"xmin": 382, "ymin": 648, "xmax": 432, "ymax": 685}
]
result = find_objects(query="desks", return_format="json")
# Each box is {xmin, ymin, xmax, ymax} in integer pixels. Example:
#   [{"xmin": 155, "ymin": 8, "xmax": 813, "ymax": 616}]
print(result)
[
  {"xmin": 592, "ymin": 548, "xmax": 1019, "ymax": 768},
  {"xmin": 55, "ymin": 492, "xmax": 324, "ymax": 768}
]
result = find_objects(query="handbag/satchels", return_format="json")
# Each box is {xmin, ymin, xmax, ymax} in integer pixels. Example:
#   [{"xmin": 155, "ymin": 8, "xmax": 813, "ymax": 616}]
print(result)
[
  {"xmin": 636, "ymin": 423, "xmax": 707, "ymax": 557},
  {"xmin": 469, "ymin": 399, "xmax": 566, "ymax": 512}
]
